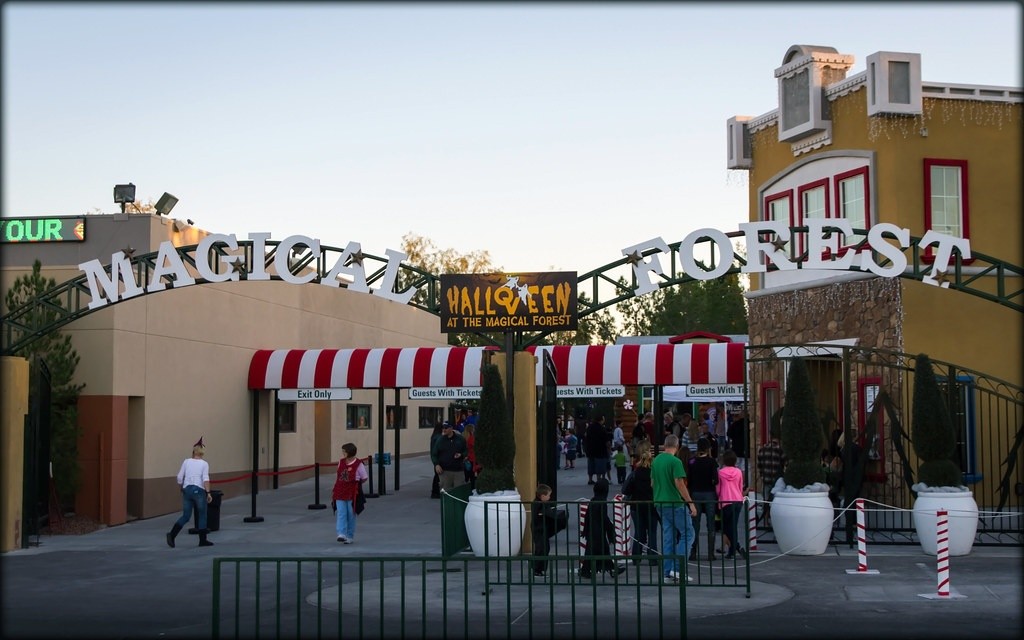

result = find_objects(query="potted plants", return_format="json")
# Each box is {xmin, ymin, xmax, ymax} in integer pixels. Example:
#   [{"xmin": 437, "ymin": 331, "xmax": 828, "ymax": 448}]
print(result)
[
  {"xmin": 768, "ymin": 359, "xmax": 834, "ymax": 556},
  {"xmin": 909, "ymin": 352, "xmax": 980, "ymax": 557},
  {"xmin": 466, "ymin": 362, "xmax": 527, "ymax": 556}
]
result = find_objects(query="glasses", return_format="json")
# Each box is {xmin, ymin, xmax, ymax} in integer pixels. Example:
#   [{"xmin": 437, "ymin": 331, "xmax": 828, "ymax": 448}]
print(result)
[{"xmin": 442, "ymin": 426, "xmax": 449, "ymax": 430}]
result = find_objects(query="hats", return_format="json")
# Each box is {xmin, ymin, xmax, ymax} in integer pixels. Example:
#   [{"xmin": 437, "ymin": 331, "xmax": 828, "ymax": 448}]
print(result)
[{"xmin": 442, "ymin": 420, "xmax": 454, "ymax": 426}]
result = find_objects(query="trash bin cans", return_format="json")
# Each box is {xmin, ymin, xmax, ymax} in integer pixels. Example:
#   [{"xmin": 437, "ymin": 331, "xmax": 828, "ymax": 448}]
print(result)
[{"xmin": 194, "ymin": 490, "xmax": 224, "ymax": 531}]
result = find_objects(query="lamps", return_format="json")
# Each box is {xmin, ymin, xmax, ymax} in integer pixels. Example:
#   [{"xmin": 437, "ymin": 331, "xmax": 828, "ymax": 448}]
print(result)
[
  {"xmin": 113, "ymin": 182, "xmax": 136, "ymax": 213},
  {"xmin": 155, "ymin": 192, "xmax": 177, "ymax": 218}
]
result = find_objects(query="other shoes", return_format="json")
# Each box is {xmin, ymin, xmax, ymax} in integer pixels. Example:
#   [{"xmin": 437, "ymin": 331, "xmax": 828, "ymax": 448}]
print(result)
[
  {"xmin": 739, "ymin": 548, "xmax": 746, "ymax": 561},
  {"xmin": 715, "ymin": 547, "xmax": 725, "ymax": 554},
  {"xmin": 534, "ymin": 573, "xmax": 549, "ymax": 578},
  {"xmin": 663, "ymin": 577, "xmax": 680, "ymax": 584},
  {"xmin": 609, "ymin": 566, "xmax": 625, "ymax": 577},
  {"xmin": 337, "ymin": 535, "xmax": 348, "ymax": 542},
  {"xmin": 668, "ymin": 570, "xmax": 693, "ymax": 581},
  {"xmin": 724, "ymin": 553, "xmax": 735, "ymax": 559},
  {"xmin": 579, "ymin": 571, "xmax": 592, "ymax": 578},
  {"xmin": 345, "ymin": 538, "xmax": 353, "ymax": 544},
  {"xmin": 431, "ymin": 494, "xmax": 441, "ymax": 499},
  {"xmin": 649, "ymin": 560, "xmax": 660, "ymax": 566}
]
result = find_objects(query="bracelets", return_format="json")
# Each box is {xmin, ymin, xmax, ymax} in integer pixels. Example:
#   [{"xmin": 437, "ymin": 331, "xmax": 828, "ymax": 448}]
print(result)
[{"xmin": 207, "ymin": 491, "xmax": 210, "ymax": 494}]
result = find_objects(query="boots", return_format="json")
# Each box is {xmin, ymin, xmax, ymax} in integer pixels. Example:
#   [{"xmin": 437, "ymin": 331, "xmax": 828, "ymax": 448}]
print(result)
[
  {"xmin": 198, "ymin": 528, "xmax": 214, "ymax": 547},
  {"xmin": 689, "ymin": 531, "xmax": 697, "ymax": 561},
  {"xmin": 167, "ymin": 524, "xmax": 182, "ymax": 548},
  {"xmin": 707, "ymin": 527, "xmax": 717, "ymax": 561}
]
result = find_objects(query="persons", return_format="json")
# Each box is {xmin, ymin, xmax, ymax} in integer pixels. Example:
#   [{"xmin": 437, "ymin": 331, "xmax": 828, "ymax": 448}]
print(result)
[
  {"xmin": 331, "ymin": 443, "xmax": 368, "ymax": 544},
  {"xmin": 758, "ymin": 429, "xmax": 868, "ymax": 528},
  {"xmin": 387, "ymin": 408, "xmax": 395, "ymax": 429},
  {"xmin": 577, "ymin": 479, "xmax": 625, "ymax": 579},
  {"xmin": 530, "ymin": 485, "xmax": 553, "ymax": 578},
  {"xmin": 166, "ymin": 446, "xmax": 214, "ymax": 548},
  {"xmin": 621, "ymin": 434, "xmax": 747, "ymax": 584},
  {"xmin": 556, "ymin": 411, "xmax": 747, "ymax": 488},
  {"xmin": 430, "ymin": 408, "xmax": 480, "ymax": 499},
  {"xmin": 358, "ymin": 416, "xmax": 368, "ymax": 428}
]
